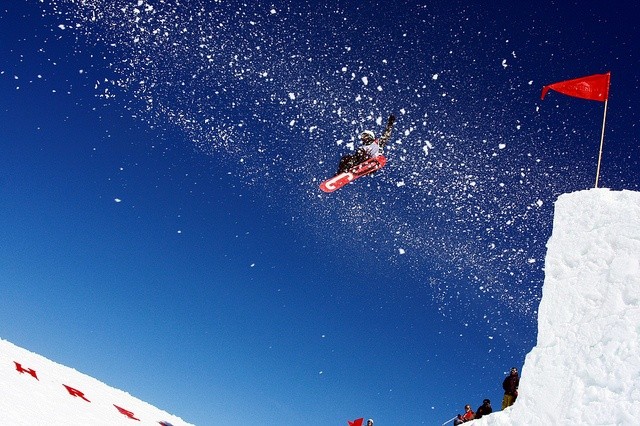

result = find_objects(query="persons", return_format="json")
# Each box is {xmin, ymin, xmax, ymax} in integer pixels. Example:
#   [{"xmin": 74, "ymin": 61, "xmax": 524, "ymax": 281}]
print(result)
[
  {"xmin": 476, "ymin": 399, "xmax": 492, "ymax": 419},
  {"xmin": 367, "ymin": 419, "xmax": 374, "ymax": 426},
  {"xmin": 501, "ymin": 367, "xmax": 519, "ymax": 410},
  {"xmin": 458, "ymin": 405, "xmax": 476, "ymax": 423},
  {"xmin": 334, "ymin": 114, "xmax": 396, "ymax": 177}
]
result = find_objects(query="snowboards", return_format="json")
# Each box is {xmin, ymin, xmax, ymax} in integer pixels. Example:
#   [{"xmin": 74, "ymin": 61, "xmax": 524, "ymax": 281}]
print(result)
[{"xmin": 320, "ymin": 156, "xmax": 386, "ymax": 193}]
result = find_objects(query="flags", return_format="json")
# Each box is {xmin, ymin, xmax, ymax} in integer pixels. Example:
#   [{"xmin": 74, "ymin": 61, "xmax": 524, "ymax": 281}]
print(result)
[
  {"xmin": 541, "ymin": 72, "xmax": 610, "ymax": 102},
  {"xmin": 348, "ymin": 418, "xmax": 363, "ymax": 425}
]
